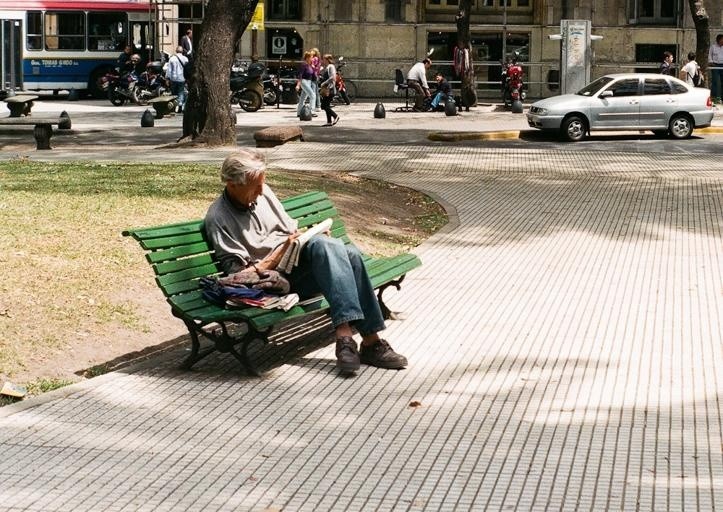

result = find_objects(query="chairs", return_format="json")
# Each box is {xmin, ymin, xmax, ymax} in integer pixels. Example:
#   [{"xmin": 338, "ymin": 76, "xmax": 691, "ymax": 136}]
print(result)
[{"xmin": 395, "ymin": 68, "xmax": 415, "ymax": 112}]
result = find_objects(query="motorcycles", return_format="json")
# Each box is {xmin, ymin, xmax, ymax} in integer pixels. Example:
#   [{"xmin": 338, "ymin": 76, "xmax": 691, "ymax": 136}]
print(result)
[
  {"xmin": 95, "ymin": 50, "xmax": 351, "ymax": 114},
  {"xmin": 499, "ymin": 51, "xmax": 528, "ymax": 114}
]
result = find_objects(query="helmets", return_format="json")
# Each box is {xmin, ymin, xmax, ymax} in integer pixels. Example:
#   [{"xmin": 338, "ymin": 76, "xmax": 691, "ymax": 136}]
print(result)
[{"xmin": 131, "ymin": 54, "xmax": 141, "ymax": 64}]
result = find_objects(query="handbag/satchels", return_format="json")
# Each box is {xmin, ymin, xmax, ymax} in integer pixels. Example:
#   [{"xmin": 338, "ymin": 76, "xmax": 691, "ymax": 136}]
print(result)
[
  {"xmin": 311, "ymin": 71, "xmax": 318, "ymax": 82},
  {"xmin": 320, "ymin": 86, "xmax": 329, "ymax": 96},
  {"xmin": 199, "ymin": 266, "xmax": 290, "ymax": 295},
  {"xmin": 423, "ymin": 97, "xmax": 439, "ymax": 111},
  {"xmin": 693, "ymin": 74, "xmax": 704, "ymax": 87}
]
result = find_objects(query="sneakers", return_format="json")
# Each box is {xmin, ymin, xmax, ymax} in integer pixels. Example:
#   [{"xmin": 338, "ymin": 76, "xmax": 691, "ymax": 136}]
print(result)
[
  {"xmin": 178, "ymin": 103, "xmax": 182, "ymax": 113},
  {"xmin": 322, "ymin": 125, "xmax": 332, "ymax": 127},
  {"xmin": 311, "ymin": 108, "xmax": 321, "ymax": 116},
  {"xmin": 333, "ymin": 117, "xmax": 339, "ymax": 125}
]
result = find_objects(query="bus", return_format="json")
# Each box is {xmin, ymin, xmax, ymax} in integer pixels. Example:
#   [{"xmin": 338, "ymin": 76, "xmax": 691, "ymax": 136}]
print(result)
[{"xmin": 0, "ymin": 0, "xmax": 158, "ymax": 97}]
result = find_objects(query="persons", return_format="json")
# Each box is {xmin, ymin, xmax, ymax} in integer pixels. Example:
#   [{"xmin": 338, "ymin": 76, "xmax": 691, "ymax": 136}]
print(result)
[
  {"xmin": 203, "ymin": 149, "xmax": 408, "ymax": 371},
  {"xmin": 660, "ymin": 52, "xmax": 673, "ymax": 75},
  {"xmin": 428, "ymin": 73, "xmax": 454, "ymax": 112},
  {"xmin": 116, "ymin": 29, "xmax": 193, "ymax": 114},
  {"xmin": 319, "ymin": 54, "xmax": 339, "ymax": 126},
  {"xmin": 708, "ymin": 34, "xmax": 723, "ymax": 97},
  {"xmin": 407, "ymin": 58, "xmax": 432, "ymax": 111},
  {"xmin": 681, "ymin": 51, "xmax": 697, "ymax": 87},
  {"xmin": 309, "ymin": 47, "xmax": 321, "ymax": 107},
  {"xmin": 294, "ymin": 51, "xmax": 317, "ymax": 117}
]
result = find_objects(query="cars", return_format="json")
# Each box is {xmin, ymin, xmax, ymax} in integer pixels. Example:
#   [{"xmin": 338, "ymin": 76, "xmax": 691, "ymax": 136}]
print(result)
[{"xmin": 526, "ymin": 73, "xmax": 714, "ymax": 142}]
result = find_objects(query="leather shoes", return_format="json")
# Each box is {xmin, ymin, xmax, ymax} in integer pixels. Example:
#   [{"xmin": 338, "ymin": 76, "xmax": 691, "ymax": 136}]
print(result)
[
  {"xmin": 360, "ymin": 340, "xmax": 407, "ymax": 368},
  {"xmin": 337, "ymin": 336, "xmax": 360, "ymax": 370}
]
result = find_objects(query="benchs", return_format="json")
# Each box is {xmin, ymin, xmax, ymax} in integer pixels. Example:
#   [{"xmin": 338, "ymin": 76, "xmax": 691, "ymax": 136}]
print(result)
[
  {"xmin": 121, "ymin": 190, "xmax": 423, "ymax": 379},
  {"xmin": 3, "ymin": 95, "xmax": 39, "ymax": 117},
  {"xmin": 0, "ymin": 118, "xmax": 69, "ymax": 150},
  {"xmin": 147, "ymin": 95, "xmax": 179, "ymax": 119}
]
